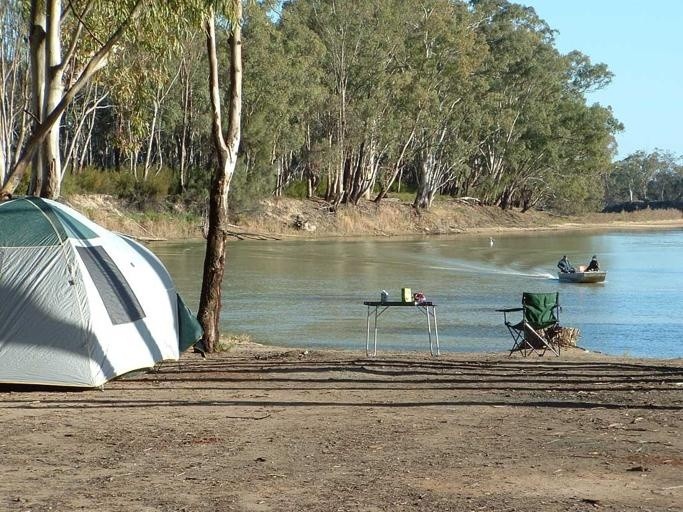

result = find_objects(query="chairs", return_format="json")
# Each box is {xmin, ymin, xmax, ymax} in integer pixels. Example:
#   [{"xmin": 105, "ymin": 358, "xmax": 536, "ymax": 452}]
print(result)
[{"xmin": 495, "ymin": 291, "xmax": 562, "ymax": 358}]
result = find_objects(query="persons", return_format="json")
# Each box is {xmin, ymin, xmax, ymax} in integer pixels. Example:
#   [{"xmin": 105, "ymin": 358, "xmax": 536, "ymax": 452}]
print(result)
[
  {"xmin": 557, "ymin": 256, "xmax": 575, "ymax": 273},
  {"xmin": 584, "ymin": 255, "xmax": 600, "ymax": 272}
]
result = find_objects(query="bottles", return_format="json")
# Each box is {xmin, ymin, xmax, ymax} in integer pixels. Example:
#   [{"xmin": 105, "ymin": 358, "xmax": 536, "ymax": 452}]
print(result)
[{"xmin": 381, "ymin": 290, "xmax": 388, "ymax": 301}]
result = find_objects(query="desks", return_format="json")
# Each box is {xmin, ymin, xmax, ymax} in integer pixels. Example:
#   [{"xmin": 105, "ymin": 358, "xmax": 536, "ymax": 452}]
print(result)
[{"xmin": 364, "ymin": 301, "xmax": 441, "ymax": 357}]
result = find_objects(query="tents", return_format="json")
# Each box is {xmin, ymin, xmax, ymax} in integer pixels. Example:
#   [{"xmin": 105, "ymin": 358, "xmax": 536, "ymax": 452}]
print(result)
[{"xmin": 0, "ymin": 195, "xmax": 204, "ymax": 391}]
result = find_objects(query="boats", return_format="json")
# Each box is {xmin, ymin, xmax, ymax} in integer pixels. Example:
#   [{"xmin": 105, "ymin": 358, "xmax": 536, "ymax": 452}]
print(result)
[{"xmin": 556, "ymin": 265, "xmax": 606, "ymax": 284}]
